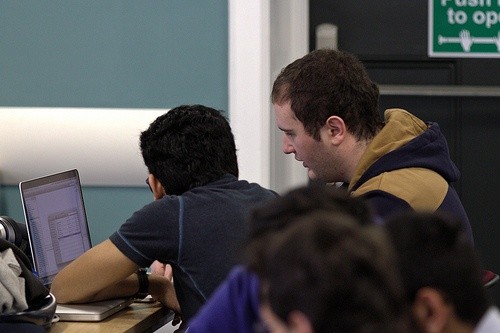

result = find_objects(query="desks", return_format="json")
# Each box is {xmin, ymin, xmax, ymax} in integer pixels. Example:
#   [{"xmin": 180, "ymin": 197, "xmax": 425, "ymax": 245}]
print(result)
[{"xmin": 46, "ymin": 291, "xmax": 178, "ymax": 333}]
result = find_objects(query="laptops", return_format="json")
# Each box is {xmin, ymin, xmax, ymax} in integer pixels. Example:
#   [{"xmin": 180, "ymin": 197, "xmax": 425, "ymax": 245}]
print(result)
[{"xmin": 19, "ymin": 169, "xmax": 136, "ymax": 320}]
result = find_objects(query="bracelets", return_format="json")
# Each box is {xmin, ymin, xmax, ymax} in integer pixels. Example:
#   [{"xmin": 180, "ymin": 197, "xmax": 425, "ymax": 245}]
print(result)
[{"xmin": 136, "ymin": 267, "xmax": 150, "ymax": 295}]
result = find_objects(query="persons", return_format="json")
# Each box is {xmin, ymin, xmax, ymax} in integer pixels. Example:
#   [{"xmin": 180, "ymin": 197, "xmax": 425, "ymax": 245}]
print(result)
[
  {"xmin": 184, "ymin": 183, "xmax": 500, "ymax": 333},
  {"xmin": 48, "ymin": 104, "xmax": 284, "ymax": 333},
  {"xmin": 270, "ymin": 48, "xmax": 475, "ymax": 257}
]
